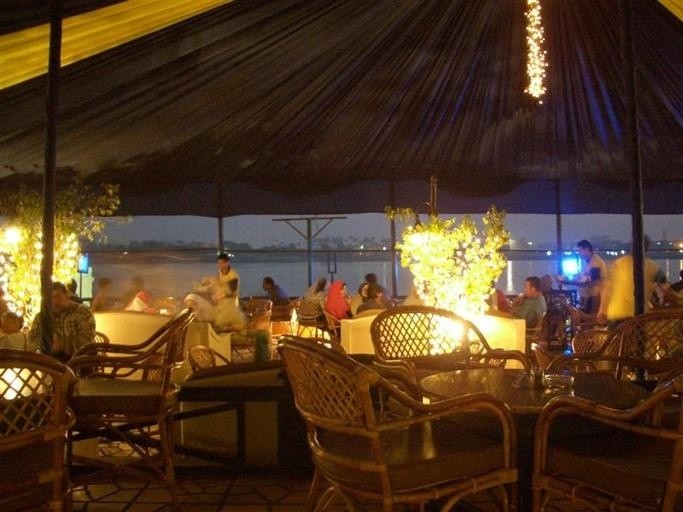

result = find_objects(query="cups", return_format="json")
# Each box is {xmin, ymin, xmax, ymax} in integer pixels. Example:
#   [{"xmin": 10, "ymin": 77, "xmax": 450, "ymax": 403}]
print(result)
[{"xmin": 530, "ymin": 367, "xmax": 545, "ymax": 386}]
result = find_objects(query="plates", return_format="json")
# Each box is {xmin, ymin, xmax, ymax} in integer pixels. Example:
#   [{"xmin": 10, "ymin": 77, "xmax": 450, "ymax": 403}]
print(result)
[{"xmin": 545, "ymin": 375, "xmax": 575, "ymax": 386}]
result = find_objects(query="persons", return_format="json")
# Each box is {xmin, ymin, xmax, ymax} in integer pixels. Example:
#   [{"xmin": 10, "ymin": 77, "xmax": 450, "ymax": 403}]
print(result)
[
  {"xmin": 1, "ymin": 255, "xmax": 426, "ymax": 378},
  {"xmin": 486, "ymin": 236, "xmax": 683, "ymax": 382}
]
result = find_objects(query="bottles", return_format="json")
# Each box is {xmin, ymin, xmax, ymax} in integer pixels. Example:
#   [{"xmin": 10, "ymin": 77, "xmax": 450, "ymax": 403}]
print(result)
[{"xmin": 565, "ymin": 320, "xmax": 573, "ymax": 346}]
